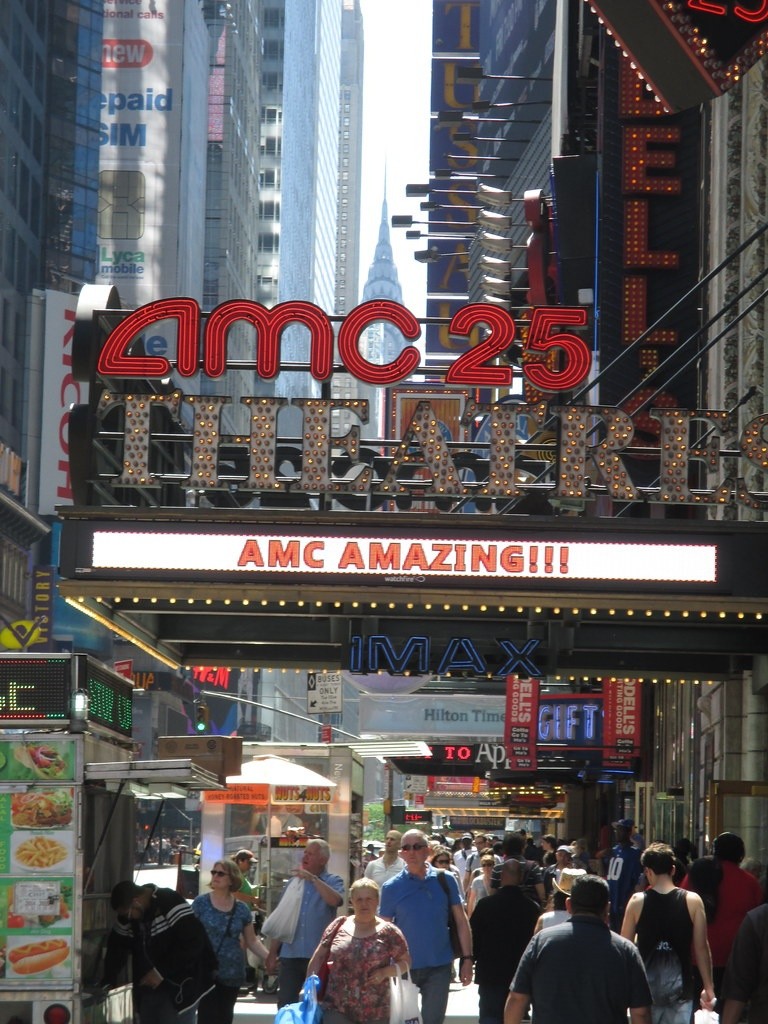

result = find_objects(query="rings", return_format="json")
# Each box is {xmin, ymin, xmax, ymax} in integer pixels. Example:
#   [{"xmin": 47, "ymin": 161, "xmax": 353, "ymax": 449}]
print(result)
[{"xmin": 376, "ymin": 977, "xmax": 377, "ymax": 979}]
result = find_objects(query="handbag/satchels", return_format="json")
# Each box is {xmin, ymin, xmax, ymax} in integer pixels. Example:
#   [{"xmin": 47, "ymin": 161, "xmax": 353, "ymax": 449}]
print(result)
[
  {"xmin": 438, "ymin": 868, "xmax": 462, "ymax": 959},
  {"xmin": 261, "ymin": 877, "xmax": 305, "ymax": 943},
  {"xmin": 694, "ymin": 1009, "xmax": 720, "ymax": 1024},
  {"xmin": 390, "ymin": 959, "xmax": 423, "ymax": 1024}
]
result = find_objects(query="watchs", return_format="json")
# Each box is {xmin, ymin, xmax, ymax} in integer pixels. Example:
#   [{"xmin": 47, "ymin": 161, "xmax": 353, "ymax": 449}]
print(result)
[{"xmin": 461, "ymin": 956, "xmax": 477, "ymax": 965}]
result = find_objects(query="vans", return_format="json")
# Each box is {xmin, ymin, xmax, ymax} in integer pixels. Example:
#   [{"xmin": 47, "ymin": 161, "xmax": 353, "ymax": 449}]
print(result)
[{"xmin": 172, "ymin": 828, "xmax": 324, "ymax": 914}]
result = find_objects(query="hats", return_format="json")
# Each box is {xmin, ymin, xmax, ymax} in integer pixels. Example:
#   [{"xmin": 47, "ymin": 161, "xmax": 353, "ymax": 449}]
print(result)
[
  {"xmin": 557, "ymin": 845, "xmax": 571, "ymax": 854},
  {"xmin": 552, "ymin": 868, "xmax": 587, "ymax": 895},
  {"xmin": 236, "ymin": 850, "xmax": 258, "ymax": 863},
  {"xmin": 462, "ymin": 831, "xmax": 474, "ymax": 840},
  {"xmin": 612, "ymin": 819, "xmax": 632, "ymax": 830}
]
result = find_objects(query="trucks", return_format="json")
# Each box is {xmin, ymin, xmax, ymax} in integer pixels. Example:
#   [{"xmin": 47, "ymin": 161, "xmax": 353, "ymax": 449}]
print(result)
[{"xmin": 0, "ymin": 644, "xmax": 229, "ymax": 1024}]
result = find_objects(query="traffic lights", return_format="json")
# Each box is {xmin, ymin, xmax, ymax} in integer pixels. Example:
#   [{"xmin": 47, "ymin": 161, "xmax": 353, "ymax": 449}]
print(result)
[{"xmin": 196, "ymin": 706, "xmax": 206, "ymax": 731}]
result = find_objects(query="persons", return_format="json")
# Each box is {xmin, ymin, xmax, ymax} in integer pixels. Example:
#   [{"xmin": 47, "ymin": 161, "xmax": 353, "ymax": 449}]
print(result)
[
  {"xmin": 189, "ymin": 839, "xmax": 346, "ymax": 1024},
  {"xmin": 365, "ymin": 829, "xmax": 408, "ymax": 923},
  {"xmin": 379, "ymin": 820, "xmax": 768, "ymax": 1024},
  {"xmin": 97, "ymin": 880, "xmax": 221, "ymax": 1024},
  {"xmin": 298, "ymin": 877, "xmax": 412, "ymax": 1024}
]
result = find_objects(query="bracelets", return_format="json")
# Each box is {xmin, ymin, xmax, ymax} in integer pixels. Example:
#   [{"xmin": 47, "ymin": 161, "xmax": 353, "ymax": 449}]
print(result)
[{"xmin": 311, "ymin": 875, "xmax": 318, "ymax": 882}]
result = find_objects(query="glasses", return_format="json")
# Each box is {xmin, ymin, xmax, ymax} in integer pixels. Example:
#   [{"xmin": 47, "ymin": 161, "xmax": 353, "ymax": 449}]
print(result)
[
  {"xmin": 435, "ymin": 859, "xmax": 450, "ymax": 865},
  {"xmin": 402, "ymin": 843, "xmax": 425, "ymax": 851},
  {"xmin": 482, "ymin": 863, "xmax": 491, "ymax": 867},
  {"xmin": 211, "ymin": 870, "xmax": 230, "ymax": 877}
]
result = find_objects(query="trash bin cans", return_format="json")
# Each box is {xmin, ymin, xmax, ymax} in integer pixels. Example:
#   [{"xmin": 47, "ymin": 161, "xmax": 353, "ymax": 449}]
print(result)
[
  {"xmin": 171, "ymin": 853, "xmax": 180, "ymax": 864},
  {"xmin": 177, "ymin": 845, "xmax": 189, "ymax": 864}
]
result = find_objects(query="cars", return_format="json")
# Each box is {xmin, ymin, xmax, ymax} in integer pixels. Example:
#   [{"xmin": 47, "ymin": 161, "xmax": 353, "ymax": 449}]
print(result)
[{"xmin": 361, "ymin": 840, "xmax": 386, "ymax": 857}]
